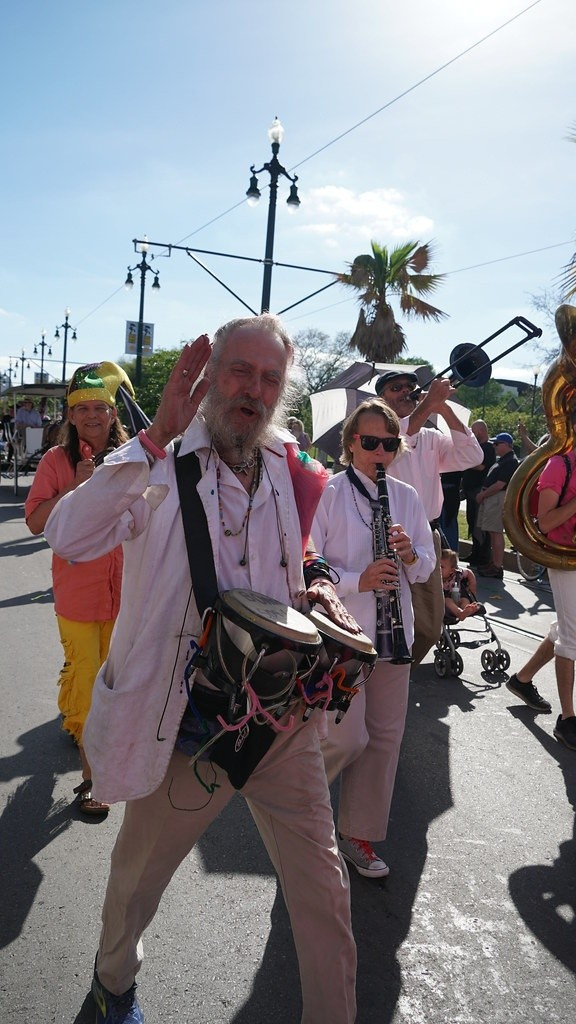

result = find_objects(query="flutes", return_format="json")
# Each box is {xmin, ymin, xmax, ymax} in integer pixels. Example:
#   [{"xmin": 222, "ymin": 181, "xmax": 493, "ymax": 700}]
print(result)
[
  {"xmin": 377, "ymin": 461, "xmax": 416, "ymax": 664},
  {"xmin": 371, "ymin": 500, "xmax": 394, "ymax": 663}
]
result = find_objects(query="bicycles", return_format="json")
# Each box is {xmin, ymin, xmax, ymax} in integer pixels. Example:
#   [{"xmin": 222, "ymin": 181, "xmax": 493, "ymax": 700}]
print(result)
[{"xmin": 517, "ymin": 548, "xmax": 546, "ymax": 581}]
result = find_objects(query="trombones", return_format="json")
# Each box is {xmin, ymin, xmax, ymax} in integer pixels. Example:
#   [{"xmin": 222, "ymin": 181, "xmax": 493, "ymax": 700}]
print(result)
[{"xmin": 404, "ymin": 315, "xmax": 543, "ymax": 402}]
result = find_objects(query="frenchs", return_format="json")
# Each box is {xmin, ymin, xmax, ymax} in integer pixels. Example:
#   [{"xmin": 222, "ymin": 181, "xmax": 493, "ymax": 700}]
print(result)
[{"xmin": 502, "ymin": 303, "xmax": 576, "ymax": 572}]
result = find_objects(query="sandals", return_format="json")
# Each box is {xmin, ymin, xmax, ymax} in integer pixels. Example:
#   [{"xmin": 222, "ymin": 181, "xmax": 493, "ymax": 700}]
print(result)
[{"xmin": 72, "ymin": 777, "xmax": 109, "ymax": 813}]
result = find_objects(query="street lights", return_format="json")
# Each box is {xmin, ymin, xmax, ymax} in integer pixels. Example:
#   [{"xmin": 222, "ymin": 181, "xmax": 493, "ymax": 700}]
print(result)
[
  {"xmin": 33, "ymin": 334, "xmax": 52, "ymax": 383},
  {"xmin": 124, "ymin": 234, "xmax": 161, "ymax": 387},
  {"xmin": 54, "ymin": 307, "xmax": 77, "ymax": 383},
  {"xmin": 16, "ymin": 350, "xmax": 30, "ymax": 386},
  {"xmin": 246, "ymin": 116, "xmax": 301, "ymax": 317},
  {"xmin": 0, "ymin": 362, "xmax": 14, "ymax": 389}
]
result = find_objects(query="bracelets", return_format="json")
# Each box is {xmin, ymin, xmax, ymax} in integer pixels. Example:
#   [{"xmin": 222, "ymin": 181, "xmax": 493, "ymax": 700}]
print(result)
[
  {"xmin": 137, "ymin": 428, "xmax": 166, "ymax": 464},
  {"xmin": 401, "ymin": 548, "xmax": 419, "ymax": 566},
  {"xmin": 304, "ymin": 549, "xmax": 341, "ymax": 589}
]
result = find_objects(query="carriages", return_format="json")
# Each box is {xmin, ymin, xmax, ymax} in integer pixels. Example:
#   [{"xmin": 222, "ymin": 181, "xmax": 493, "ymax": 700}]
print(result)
[{"xmin": 0, "ymin": 383, "xmax": 69, "ymax": 496}]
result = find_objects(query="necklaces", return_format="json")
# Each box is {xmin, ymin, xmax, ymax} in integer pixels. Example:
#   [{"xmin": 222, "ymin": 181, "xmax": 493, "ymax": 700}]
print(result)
[
  {"xmin": 347, "ymin": 475, "xmax": 372, "ymax": 530},
  {"xmin": 409, "ymin": 437, "xmax": 418, "ymax": 449},
  {"xmin": 215, "ymin": 448, "xmax": 257, "ymax": 537}
]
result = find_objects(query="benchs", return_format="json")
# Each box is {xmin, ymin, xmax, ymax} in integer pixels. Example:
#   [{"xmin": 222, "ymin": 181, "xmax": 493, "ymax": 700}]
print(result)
[{"xmin": 0, "ymin": 421, "xmax": 24, "ymax": 446}]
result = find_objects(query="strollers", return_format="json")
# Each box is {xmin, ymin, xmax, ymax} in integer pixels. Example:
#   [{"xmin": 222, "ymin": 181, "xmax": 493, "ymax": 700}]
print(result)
[{"xmin": 433, "ymin": 524, "xmax": 511, "ymax": 678}]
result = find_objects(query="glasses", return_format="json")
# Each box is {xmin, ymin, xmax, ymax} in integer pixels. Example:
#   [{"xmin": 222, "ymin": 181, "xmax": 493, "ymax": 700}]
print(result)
[
  {"xmin": 376, "ymin": 383, "xmax": 417, "ymax": 397},
  {"xmin": 351, "ymin": 434, "xmax": 401, "ymax": 453},
  {"xmin": 39, "ymin": 411, "xmax": 45, "ymax": 414},
  {"xmin": 493, "ymin": 441, "xmax": 505, "ymax": 446}
]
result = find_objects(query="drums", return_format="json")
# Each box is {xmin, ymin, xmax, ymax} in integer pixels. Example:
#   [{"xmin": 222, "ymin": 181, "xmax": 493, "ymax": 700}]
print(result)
[
  {"xmin": 301, "ymin": 607, "xmax": 378, "ymax": 712},
  {"xmin": 192, "ymin": 586, "xmax": 323, "ymax": 702}
]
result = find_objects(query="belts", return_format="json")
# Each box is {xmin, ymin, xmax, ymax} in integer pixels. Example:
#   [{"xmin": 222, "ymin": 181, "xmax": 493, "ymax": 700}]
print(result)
[{"xmin": 482, "ymin": 488, "xmax": 487, "ymax": 491}]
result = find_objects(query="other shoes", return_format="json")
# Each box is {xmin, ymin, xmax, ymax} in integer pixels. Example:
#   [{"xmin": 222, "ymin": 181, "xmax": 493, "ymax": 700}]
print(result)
[
  {"xmin": 459, "ymin": 553, "xmax": 474, "ymax": 562},
  {"xmin": 477, "ymin": 561, "xmax": 504, "ymax": 579},
  {"xmin": 90, "ymin": 976, "xmax": 145, "ymax": 1024},
  {"xmin": 467, "ymin": 562, "xmax": 490, "ymax": 571}
]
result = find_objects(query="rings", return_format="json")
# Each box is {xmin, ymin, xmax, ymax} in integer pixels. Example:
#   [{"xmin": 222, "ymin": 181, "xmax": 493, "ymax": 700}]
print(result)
[{"xmin": 182, "ymin": 369, "xmax": 189, "ymax": 376}]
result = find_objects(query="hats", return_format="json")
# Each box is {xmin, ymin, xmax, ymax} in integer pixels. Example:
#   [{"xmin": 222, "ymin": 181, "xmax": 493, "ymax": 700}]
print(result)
[
  {"xmin": 67, "ymin": 361, "xmax": 136, "ymax": 409},
  {"xmin": 22, "ymin": 396, "xmax": 34, "ymax": 404},
  {"xmin": 8, "ymin": 404, "xmax": 21, "ymax": 411},
  {"xmin": 374, "ymin": 369, "xmax": 419, "ymax": 393},
  {"xmin": 489, "ymin": 433, "xmax": 513, "ymax": 444}
]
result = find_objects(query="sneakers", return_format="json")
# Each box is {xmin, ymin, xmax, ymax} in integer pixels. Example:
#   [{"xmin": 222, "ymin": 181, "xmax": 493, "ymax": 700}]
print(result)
[
  {"xmin": 553, "ymin": 714, "xmax": 576, "ymax": 751},
  {"xmin": 335, "ymin": 831, "xmax": 389, "ymax": 878},
  {"xmin": 506, "ymin": 671, "xmax": 551, "ymax": 710}
]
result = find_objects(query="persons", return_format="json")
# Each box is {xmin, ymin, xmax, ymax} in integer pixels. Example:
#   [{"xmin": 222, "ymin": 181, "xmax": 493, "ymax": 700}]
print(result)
[
  {"xmin": 288, "ymin": 416, "xmax": 313, "ymax": 450},
  {"xmin": 25, "ymin": 360, "xmax": 135, "ymax": 815},
  {"xmin": 302, "ymin": 395, "xmax": 436, "ymax": 878},
  {"xmin": 376, "ymin": 372, "xmax": 484, "ymax": 672},
  {"xmin": 440, "ymin": 472, "xmax": 461, "ymax": 554},
  {"xmin": 439, "ymin": 550, "xmax": 479, "ymax": 622},
  {"xmin": 459, "ymin": 419, "xmax": 519, "ymax": 580},
  {"xmin": 0, "ymin": 395, "xmax": 42, "ymax": 463},
  {"xmin": 506, "ymin": 408, "xmax": 576, "ymax": 751},
  {"xmin": 38, "ymin": 407, "xmax": 62, "ymax": 422},
  {"xmin": 44, "ymin": 310, "xmax": 357, "ymax": 1024}
]
